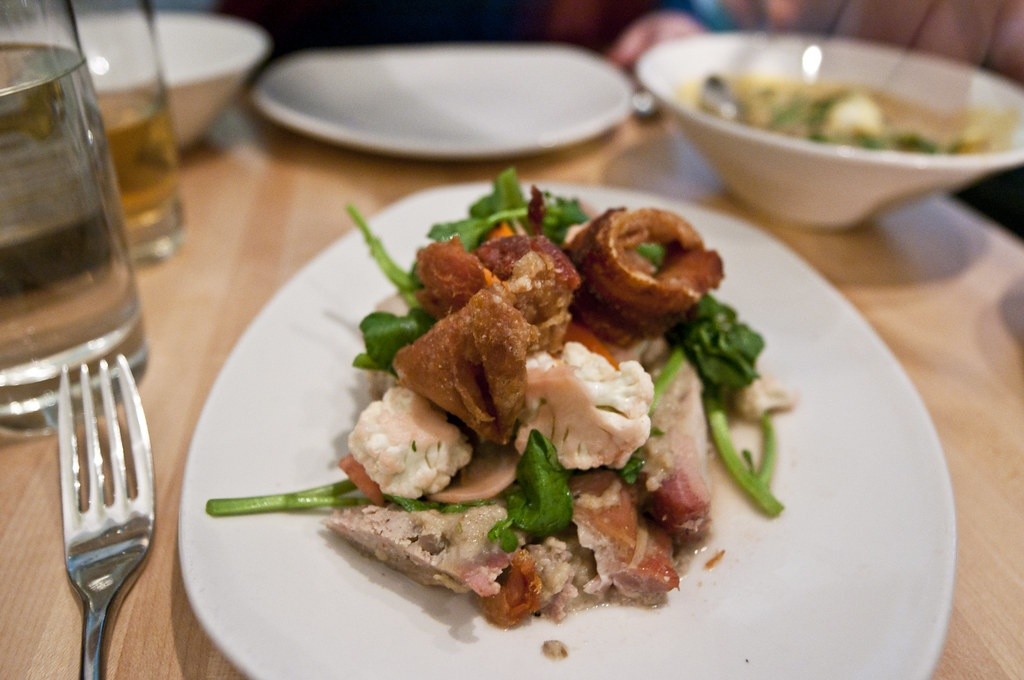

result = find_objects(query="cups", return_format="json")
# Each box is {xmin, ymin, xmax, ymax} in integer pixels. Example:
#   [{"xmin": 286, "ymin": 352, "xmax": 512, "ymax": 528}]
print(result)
[
  {"xmin": 0, "ymin": 0, "xmax": 151, "ymax": 445},
  {"xmin": 69, "ymin": 0, "xmax": 189, "ymax": 265}
]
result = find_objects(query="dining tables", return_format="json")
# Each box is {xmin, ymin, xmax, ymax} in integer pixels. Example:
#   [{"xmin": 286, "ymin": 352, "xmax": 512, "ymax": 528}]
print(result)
[{"xmin": 0, "ymin": 88, "xmax": 1024, "ymax": 680}]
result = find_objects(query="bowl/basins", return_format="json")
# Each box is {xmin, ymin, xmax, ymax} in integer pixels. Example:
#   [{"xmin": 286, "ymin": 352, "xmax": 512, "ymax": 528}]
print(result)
[
  {"xmin": 0, "ymin": 11, "xmax": 270, "ymax": 155},
  {"xmin": 633, "ymin": 32, "xmax": 1024, "ymax": 232}
]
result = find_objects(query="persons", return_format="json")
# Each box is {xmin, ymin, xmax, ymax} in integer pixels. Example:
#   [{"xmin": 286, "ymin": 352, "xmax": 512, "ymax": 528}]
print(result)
[{"xmin": 218, "ymin": 0, "xmax": 739, "ymax": 72}]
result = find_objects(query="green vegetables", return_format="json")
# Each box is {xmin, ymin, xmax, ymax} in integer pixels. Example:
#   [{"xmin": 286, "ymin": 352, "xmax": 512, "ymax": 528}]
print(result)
[
  {"xmin": 763, "ymin": 85, "xmax": 964, "ymax": 151},
  {"xmin": 204, "ymin": 170, "xmax": 781, "ymax": 551}
]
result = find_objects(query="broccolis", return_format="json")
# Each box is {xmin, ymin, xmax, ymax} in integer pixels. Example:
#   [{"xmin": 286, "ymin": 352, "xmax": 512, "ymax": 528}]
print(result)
[
  {"xmin": 347, "ymin": 382, "xmax": 472, "ymax": 504},
  {"xmin": 516, "ymin": 340, "xmax": 654, "ymax": 470}
]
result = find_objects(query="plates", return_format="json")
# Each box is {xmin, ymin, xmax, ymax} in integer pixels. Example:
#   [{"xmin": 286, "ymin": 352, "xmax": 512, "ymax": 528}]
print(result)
[
  {"xmin": 249, "ymin": 42, "xmax": 632, "ymax": 164},
  {"xmin": 177, "ymin": 181, "xmax": 958, "ymax": 680}
]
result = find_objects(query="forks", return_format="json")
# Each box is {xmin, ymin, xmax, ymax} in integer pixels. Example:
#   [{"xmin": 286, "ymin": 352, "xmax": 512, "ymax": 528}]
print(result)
[{"xmin": 57, "ymin": 354, "xmax": 154, "ymax": 680}]
